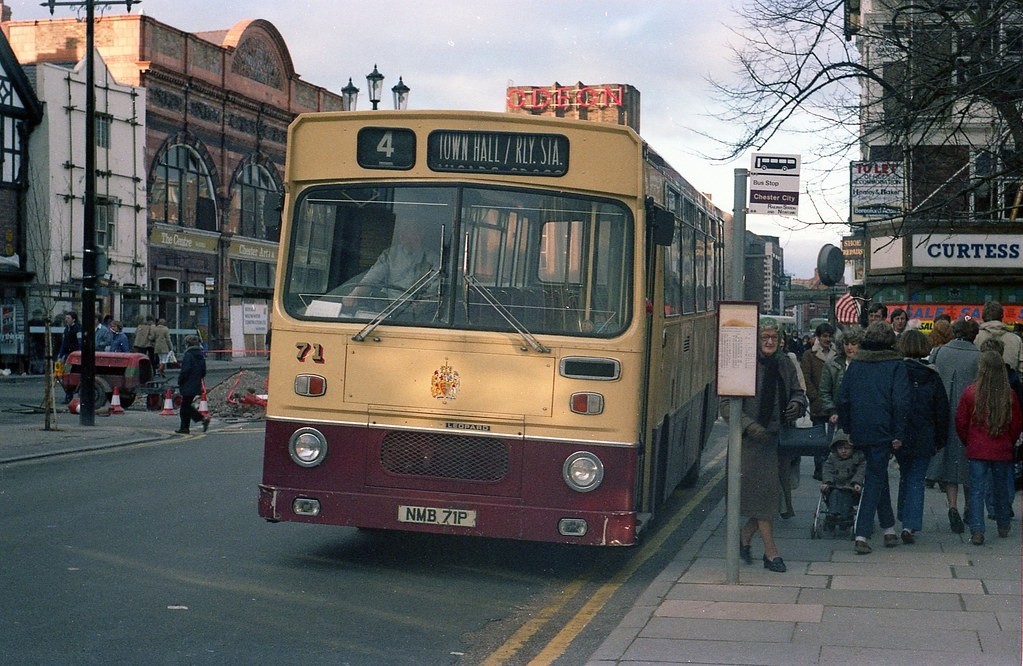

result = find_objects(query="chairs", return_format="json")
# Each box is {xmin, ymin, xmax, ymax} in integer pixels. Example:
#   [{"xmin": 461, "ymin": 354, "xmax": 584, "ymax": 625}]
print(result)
[{"xmin": 467, "ymin": 285, "xmax": 605, "ymax": 332}]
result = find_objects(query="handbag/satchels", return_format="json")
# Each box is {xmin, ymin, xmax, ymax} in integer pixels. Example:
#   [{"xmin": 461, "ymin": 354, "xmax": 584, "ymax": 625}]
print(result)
[
  {"xmin": 165, "ymin": 351, "xmax": 177, "ymax": 363},
  {"xmin": 779, "ymin": 405, "xmax": 829, "ymax": 456}
]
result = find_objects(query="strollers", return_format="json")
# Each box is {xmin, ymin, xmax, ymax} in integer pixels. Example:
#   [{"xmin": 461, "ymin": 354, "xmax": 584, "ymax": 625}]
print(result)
[{"xmin": 810, "ymin": 420, "xmax": 876, "ymax": 540}]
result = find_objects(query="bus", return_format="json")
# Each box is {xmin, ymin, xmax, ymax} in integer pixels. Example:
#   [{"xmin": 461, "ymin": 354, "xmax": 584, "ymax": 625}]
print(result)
[
  {"xmin": 808, "ymin": 319, "xmax": 829, "ymax": 336},
  {"xmin": 258, "ymin": 112, "xmax": 724, "ymax": 544},
  {"xmin": 759, "ymin": 314, "xmax": 796, "ymax": 336}
]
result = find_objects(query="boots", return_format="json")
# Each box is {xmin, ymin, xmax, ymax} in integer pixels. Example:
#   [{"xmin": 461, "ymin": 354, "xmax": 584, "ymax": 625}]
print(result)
[{"xmin": 157, "ymin": 364, "xmax": 165, "ymax": 377}]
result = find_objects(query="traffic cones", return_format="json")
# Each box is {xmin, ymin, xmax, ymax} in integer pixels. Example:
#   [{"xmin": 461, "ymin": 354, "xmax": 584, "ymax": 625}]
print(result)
[
  {"xmin": 198, "ymin": 391, "xmax": 212, "ymax": 418},
  {"xmin": 159, "ymin": 388, "xmax": 178, "ymax": 415},
  {"xmin": 68, "ymin": 398, "xmax": 81, "ymax": 414},
  {"xmin": 109, "ymin": 386, "xmax": 125, "ymax": 415}
]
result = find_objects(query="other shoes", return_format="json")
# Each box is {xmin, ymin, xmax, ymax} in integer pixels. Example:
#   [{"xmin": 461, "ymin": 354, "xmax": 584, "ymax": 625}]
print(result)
[
  {"xmin": 60, "ymin": 394, "xmax": 73, "ymax": 404},
  {"xmin": 823, "ymin": 511, "xmax": 850, "ymax": 533},
  {"xmin": 174, "ymin": 428, "xmax": 189, "ymax": 434},
  {"xmin": 854, "ymin": 541, "xmax": 872, "ymax": 555},
  {"xmin": 971, "ymin": 533, "xmax": 985, "ymax": 545},
  {"xmin": 202, "ymin": 419, "xmax": 210, "ymax": 432},
  {"xmin": 901, "ymin": 530, "xmax": 913, "ymax": 543},
  {"xmin": 998, "ymin": 524, "xmax": 1011, "ymax": 538},
  {"xmin": 884, "ymin": 534, "xmax": 899, "ymax": 546}
]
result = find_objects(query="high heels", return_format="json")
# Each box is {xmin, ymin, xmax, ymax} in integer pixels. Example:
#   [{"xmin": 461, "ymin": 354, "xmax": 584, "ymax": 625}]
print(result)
[{"xmin": 948, "ymin": 507, "xmax": 965, "ymax": 533}]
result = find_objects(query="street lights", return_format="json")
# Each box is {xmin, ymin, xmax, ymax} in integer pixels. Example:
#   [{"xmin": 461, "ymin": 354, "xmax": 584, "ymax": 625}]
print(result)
[{"xmin": 38, "ymin": 0, "xmax": 143, "ymax": 424}]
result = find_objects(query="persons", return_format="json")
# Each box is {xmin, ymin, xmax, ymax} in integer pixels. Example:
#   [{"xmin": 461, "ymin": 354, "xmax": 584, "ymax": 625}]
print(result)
[
  {"xmin": 264, "ymin": 322, "xmax": 272, "ymax": 360},
  {"xmin": 191, "ymin": 319, "xmax": 209, "ymax": 358},
  {"xmin": 341, "ymin": 213, "xmax": 441, "ymax": 324},
  {"xmin": 51, "ymin": 314, "xmax": 64, "ymax": 362},
  {"xmin": 779, "ymin": 300, "xmax": 1023, "ymax": 554},
  {"xmin": 56, "ymin": 310, "xmax": 83, "ymax": 404},
  {"xmin": 175, "ymin": 335, "xmax": 210, "ymax": 434},
  {"xmin": 27, "ymin": 310, "xmax": 46, "ymax": 375},
  {"xmin": 92, "ymin": 313, "xmax": 129, "ymax": 410},
  {"xmin": 665, "ymin": 272, "xmax": 694, "ymax": 315},
  {"xmin": 721, "ymin": 318, "xmax": 808, "ymax": 574},
  {"xmin": 133, "ymin": 315, "xmax": 174, "ymax": 379}
]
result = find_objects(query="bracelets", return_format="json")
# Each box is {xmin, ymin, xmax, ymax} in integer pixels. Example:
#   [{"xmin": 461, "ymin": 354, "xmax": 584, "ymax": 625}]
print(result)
[{"xmin": 349, "ymin": 293, "xmax": 356, "ymax": 296}]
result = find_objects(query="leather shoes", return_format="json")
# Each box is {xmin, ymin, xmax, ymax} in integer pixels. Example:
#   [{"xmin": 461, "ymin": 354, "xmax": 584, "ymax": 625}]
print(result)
[
  {"xmin": 738, "ymin": 528, "xmax": 757, "ymax": 564},
  {"xmin": 763, "ymin": 554, "xmax": 786, "ymax": 571}
]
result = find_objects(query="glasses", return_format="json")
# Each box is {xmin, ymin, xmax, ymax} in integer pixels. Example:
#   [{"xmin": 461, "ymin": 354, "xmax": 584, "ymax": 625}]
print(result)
[{"xmin": 760, "ymin": 334, "xmax": 779, "ymax": 341}]
